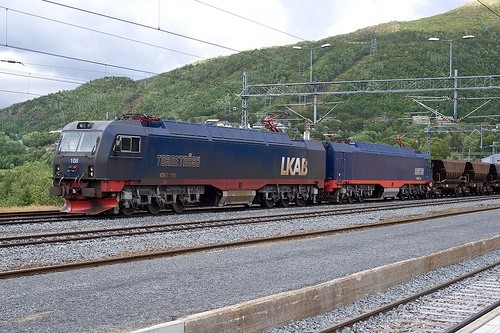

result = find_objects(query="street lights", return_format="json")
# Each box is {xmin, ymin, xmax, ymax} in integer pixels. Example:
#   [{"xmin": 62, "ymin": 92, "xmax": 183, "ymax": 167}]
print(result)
[
  {"xmin": 292, "ymin": 44, "xmax": 331, "ymax": 82},
  {"xmin": 428, "ymin": 35, "xmax": 475, "ymax": 77}
]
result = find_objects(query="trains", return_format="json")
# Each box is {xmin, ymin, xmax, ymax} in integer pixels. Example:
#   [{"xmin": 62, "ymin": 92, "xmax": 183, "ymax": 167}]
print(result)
[{"xmin": 50, "ymin": 113, "xmax": 500, "ymax": 216}]
